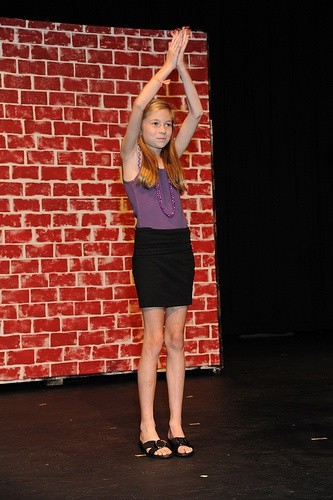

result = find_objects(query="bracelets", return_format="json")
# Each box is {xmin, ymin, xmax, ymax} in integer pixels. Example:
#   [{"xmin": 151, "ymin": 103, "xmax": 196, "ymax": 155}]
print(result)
[{"xmin": 153, "ymin": 75, "xmax": 171, "ymax": 88}]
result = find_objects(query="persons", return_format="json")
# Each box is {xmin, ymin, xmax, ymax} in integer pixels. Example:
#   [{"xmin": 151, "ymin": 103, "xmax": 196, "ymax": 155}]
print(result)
[{"xmin": 120, "ymin": 26, "xmax": 203, "ymax": 458}]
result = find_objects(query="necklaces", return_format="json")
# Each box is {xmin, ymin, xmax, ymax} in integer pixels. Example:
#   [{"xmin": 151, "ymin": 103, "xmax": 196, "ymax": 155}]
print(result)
[{"xmin": 156, "ymin": 179, "xmax": 176, "ymax": 218}]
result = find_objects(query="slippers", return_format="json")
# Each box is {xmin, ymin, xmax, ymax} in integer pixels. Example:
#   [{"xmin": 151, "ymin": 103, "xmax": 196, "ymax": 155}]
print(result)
[
  {"xmin": 138, "ymin": 438, "xmax": 173, "ymax": 459},
  {"xmin": 167, "ymin": 436, "xmax": 195, "ymax": 458}
]
